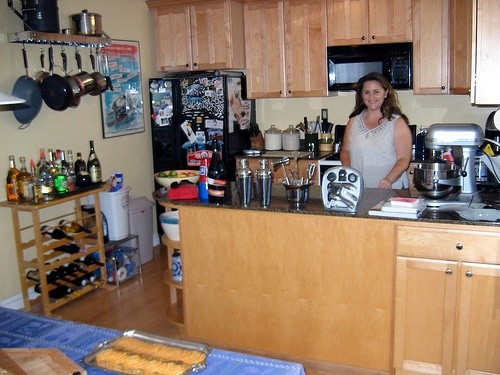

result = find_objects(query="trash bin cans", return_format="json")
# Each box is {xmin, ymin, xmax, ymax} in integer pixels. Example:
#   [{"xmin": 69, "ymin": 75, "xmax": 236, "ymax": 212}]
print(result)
[{"xmin": 126, "ymin": 195, "xmax": 155, "ymax": 267}]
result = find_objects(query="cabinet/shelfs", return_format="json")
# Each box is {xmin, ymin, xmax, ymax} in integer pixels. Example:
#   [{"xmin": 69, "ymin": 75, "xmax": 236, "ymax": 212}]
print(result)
[
  {"xmin": 0, "ymin": 182, "xmax": 112, "ymax": 317},
  {"xmin": 145, "ymin": 0, "xmax": 471, "ymax": 100},
  {"xmin": 394, "ymin": 224, "xmax": 500, "ymax": 375}
]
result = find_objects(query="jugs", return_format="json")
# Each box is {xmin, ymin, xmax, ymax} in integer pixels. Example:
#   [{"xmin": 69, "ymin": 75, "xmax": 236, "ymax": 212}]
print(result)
[{"xmin": 73, "ymin": 203, "xmax": 109, "ymax": 244}]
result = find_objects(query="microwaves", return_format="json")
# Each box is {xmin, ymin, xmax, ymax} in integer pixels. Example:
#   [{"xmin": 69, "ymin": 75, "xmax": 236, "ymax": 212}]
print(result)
[{"xmin": 326, "ymin": 41, "xmax": 412, "ymax": 92}]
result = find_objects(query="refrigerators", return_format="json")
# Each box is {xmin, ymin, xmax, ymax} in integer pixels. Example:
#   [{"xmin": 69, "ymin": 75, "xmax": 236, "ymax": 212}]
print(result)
[{"xmin": 149, "ymin": 71, "xmax": 256, "ymax": 242}]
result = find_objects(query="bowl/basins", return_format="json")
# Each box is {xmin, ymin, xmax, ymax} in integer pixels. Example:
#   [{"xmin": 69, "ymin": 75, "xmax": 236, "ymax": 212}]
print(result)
[
  {"xmin": 159, "ymin": 212, "xmax": 179, "ymax": 242},
  {"xmin": 155, "ymin": 170, "xmax": 200, "ymax": 186},
  {"xmin": 413, "ymin": 162, "xmax": 459, "ymax": 199}
]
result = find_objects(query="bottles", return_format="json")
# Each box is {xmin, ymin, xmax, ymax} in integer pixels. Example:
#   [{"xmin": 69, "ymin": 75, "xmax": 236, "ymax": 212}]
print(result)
[
  {"xmin": 207, "ymin": 139, "xmax": 230, "ymax": 205},
  {"xmin": 106, "ymin": 171, "xmax": 125, "ymax": 192},
  {"xmin": 172, "ymin": 248, "xmax": 182, "ymax": 281},
  {"xmin": 264, "ymin": 123, "xmax": 281, "ymax": 151},
  {"xmin": 194, "ymin": 116, "xmax": 206, "ymax": 144},
  {"xmin": 255, "ymin": 159, "xmax": 272, "ymax": 208},
  {"xmin": 235, "ymin": 158, "xmax": 253, "ymax": 207},
  {"xmin": 28, "ymin": 218, "xmax": 106, "ymax": 300},
  {"xmin": 281, "ymin": 122, "xmax": 301, "ymax": 150},
  {"xmin": 416, "ymin": 131, "xmax": 431, "ymax": 163},
  {"xmin": 7, "ymin": 139, "xmax": 102, "ymax": 204}
]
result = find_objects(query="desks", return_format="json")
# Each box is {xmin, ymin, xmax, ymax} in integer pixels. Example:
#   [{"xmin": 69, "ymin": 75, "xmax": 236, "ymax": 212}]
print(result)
[{"xmin": 0, "ymin": 308, "xmax": 304, "ymax": 375}]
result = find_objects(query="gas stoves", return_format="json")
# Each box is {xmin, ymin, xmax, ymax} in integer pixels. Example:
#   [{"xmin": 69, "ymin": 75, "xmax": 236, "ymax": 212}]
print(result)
[{"xmin": 317, "ymin": 124, "xmax": 417, "ymax": 162}]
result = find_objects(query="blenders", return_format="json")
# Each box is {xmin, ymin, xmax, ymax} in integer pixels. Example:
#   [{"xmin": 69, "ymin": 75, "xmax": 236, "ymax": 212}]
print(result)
[{"xmin": 408, "ymin": 121, "xmax": 482, "ymax": 207}]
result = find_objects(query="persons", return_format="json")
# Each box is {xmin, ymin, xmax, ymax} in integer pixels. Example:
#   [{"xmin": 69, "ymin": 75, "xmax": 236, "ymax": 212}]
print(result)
[{"xmin": 340, "ymin": 73, "xmax": 413, "ymax": 190}]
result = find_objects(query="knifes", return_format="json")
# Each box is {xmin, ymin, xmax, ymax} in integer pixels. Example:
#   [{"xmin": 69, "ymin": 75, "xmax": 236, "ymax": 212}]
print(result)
[{"xmin": 249, "ymin": 122, "xmax": 260, "ymax": 137}]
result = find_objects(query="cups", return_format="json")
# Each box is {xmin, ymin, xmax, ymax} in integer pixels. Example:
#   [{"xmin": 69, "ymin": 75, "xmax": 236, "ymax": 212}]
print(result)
[
  {"xmin": 320, "ymin": 134, "xmax": 332, "ymax": 151},
  {"xmin": 281, "ymin": 179, "xmax": 312, "ymax": 204},
  {"xmin": 199, "ymin": 157, "xmax": 210, "ymax": 198},
  {"xmin": 304, "ymin": 132, "xmax": 318, "ymax": 153}
]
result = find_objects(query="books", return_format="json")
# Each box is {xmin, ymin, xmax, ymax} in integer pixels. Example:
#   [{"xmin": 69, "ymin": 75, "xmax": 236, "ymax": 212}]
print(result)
[{"xmin": 368, "ymin": 197, "xmax": 427, "ymax": 219}]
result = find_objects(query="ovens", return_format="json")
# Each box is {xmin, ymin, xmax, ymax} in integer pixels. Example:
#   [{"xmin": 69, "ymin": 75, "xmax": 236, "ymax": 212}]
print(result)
[{"xmin": 317, "ymin": 162, "xmax": 343, "ymax": 185}]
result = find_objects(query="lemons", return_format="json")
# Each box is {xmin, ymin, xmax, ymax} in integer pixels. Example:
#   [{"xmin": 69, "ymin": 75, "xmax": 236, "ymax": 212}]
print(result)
[{"xmin": 158, "ymin": 171, "xmax": 179, "ymax": 178}]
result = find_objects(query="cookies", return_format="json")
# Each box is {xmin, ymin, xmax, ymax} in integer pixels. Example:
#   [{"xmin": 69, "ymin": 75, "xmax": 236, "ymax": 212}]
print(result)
[{"xmin": 95, "ymin": 337, "xmax": 206, "ymax": 375}]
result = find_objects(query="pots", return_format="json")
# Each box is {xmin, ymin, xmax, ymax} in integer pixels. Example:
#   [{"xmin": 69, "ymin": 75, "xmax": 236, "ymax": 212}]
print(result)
[{"xmin": 7, "ymin": 0, "xmax": 114, "ymax": 132}]
